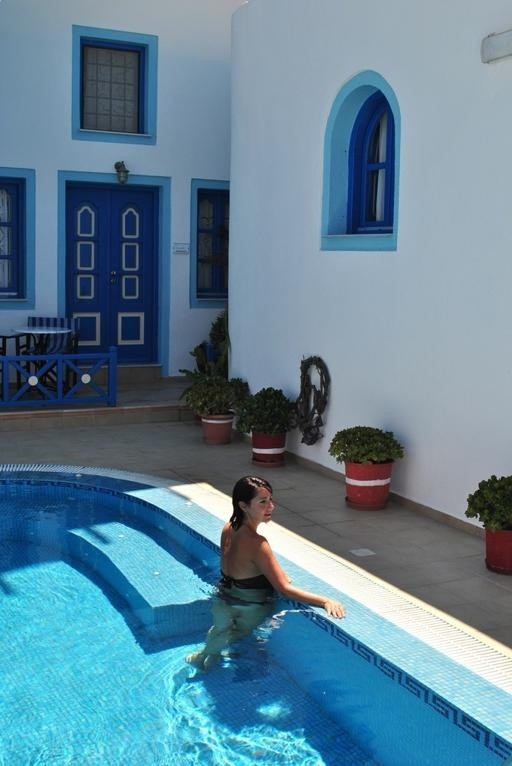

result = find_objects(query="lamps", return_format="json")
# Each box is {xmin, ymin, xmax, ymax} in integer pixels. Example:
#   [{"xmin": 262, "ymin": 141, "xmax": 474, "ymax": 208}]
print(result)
[{"xmin": 114, "ymin": 161, "xmax": 130, "ymax": 184}]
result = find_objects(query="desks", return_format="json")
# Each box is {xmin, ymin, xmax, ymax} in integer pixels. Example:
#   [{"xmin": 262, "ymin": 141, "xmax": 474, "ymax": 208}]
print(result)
[{"xmin": 0, "ymin": 325, "xmax": 71, "ymax": 401}]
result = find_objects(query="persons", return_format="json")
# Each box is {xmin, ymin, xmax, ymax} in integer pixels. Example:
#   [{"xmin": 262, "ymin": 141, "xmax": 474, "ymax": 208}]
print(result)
[{"xmin": 186, "ymin": 476, "xmax": 347, "ymax": 673}]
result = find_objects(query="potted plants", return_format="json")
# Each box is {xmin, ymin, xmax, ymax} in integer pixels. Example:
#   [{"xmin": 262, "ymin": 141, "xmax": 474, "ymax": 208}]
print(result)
[
  {"xmin": 464, "ymin": 473, "xmax": 512, "ymax": 576},
  {"xmin": 179, "ymin": 370, "xmax": 403, "ymax": 511}
]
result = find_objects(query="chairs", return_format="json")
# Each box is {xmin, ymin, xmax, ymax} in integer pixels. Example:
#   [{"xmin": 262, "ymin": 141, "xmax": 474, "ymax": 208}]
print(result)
[{"xmin": 25, "ymin": 317, "xmax": 81, "ymax": 396}]
